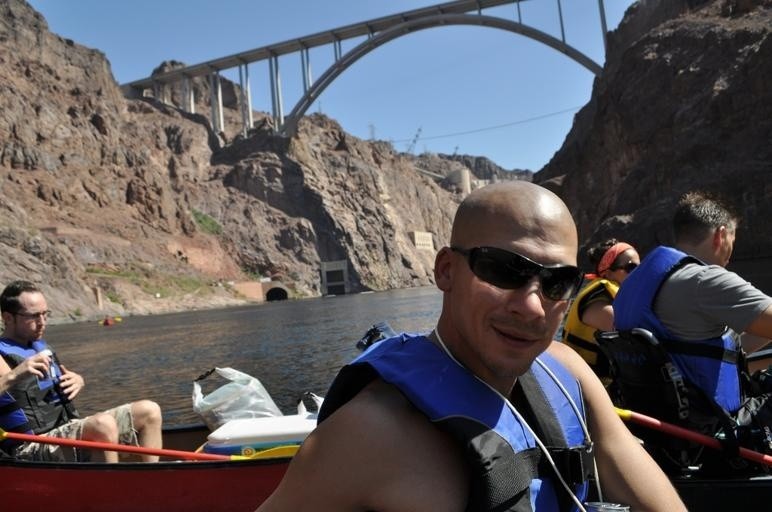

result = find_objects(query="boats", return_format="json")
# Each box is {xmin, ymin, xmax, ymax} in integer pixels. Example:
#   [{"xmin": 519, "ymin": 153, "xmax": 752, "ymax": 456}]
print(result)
[
  {"xmin": 104, "ymin": 319, "xmax": 113, "ymax": 326},
  {"xmin": 0, "ymin": 410, "xmax": 320, "ymax": 512}
]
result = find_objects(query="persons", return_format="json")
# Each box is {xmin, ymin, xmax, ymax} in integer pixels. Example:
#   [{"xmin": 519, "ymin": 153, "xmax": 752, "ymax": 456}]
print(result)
[
  {"xmin": 561, "ymin": 241, "xmax": 641, "ymax": 393},
  {"xmin": 612, "ymin": 194, "xmax": 772, "ymax": 477},
  {"xmin": 252, "ymin": 180, "xmax": 690, "ymax": 511},
  {"xmin": 0, "ymin": 280, "xmax": 165, "ymax": 463}
]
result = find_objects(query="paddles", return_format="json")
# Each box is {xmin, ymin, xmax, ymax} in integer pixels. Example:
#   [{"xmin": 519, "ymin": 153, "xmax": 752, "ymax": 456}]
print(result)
[{"xmin": 0, "ymin": 428, "xmax": 301, "ymax": 460}]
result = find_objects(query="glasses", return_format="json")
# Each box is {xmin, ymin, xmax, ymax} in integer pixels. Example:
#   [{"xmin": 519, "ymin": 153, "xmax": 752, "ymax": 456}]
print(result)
[
  {"xmin": 449, "ymin": 245, "xmax": 585, "ymax": 301},
  {"xmin": 598, "ymin": 260, "xmax": 639, "ymax": 274},
  {"xmin": 16, "ymin": 309, "xmax": 52, "ymax": 325}
]
